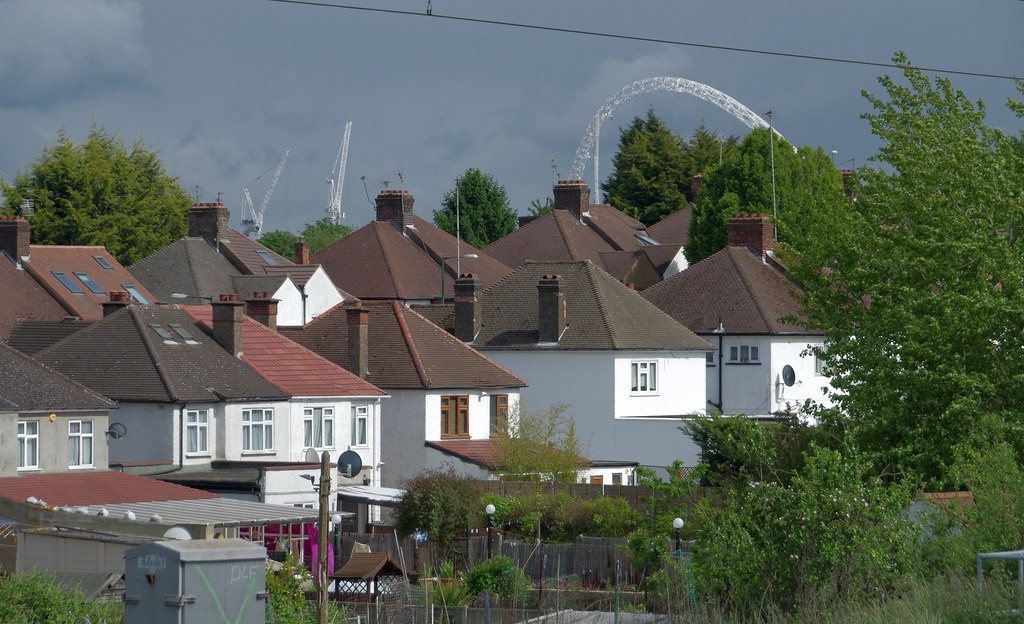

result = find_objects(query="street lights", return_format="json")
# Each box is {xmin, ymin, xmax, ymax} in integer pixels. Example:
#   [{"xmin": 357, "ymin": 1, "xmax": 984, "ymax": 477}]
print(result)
[
  {"xmin": 486, "ymin": 503, "xmax": 496, "ymax": 559},
  {"xmin": 330, "ymin": 514, "xmax": 342, "ymax": 600},
  {"xmin": 671, "ymin": 517, "xmax": 684, "ymax": 559}
]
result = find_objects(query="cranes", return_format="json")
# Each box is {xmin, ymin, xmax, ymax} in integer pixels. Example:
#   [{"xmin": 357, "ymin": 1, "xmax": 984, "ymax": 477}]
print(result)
[
  {"xmin": 240, "ymin": 149, "xmax": 293, "ymax": 238},
  {"xmin": 324, "ymin": 116, "xmax": 355, "ymax": 224}
]
